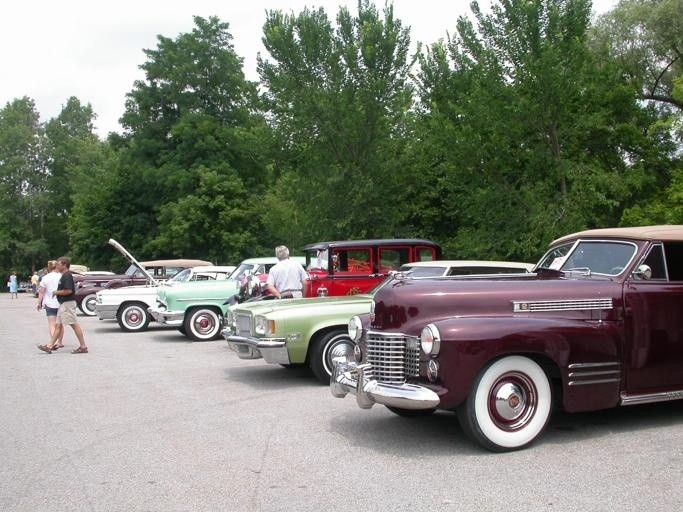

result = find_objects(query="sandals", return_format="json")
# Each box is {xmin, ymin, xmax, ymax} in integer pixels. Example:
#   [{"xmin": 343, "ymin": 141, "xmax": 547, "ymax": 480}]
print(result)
[
  {"xmin": 71, "ymin": 346, "xmax": 88, "ymax": 353},
  {"xmin": 37, "ymin": 343, "xmax": 64, "ymax": 354}
]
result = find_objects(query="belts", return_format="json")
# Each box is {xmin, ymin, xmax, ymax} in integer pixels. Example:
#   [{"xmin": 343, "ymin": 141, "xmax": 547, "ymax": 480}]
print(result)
[{"xmin": 280, "ymin": 289, "xmax": 303, "ymax": 292}]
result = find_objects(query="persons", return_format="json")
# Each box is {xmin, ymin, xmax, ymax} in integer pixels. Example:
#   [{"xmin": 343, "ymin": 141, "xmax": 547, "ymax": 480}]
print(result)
[
  {"xmin": 31, "ymin": 271, "xmax": 39, "ymax": 298},
  {"xmin": 37, "ymin": 259, "xmax": 65, "ymax": 351},
  {"xmin": 8, "ymin": 270, "xmax": 18, "ymax": 299},
  {"xmin": 333, "ymin": 253, "xmax": 341, "ymax": 272},
  {"xmin": 36, "ymin": 267, "xmax": 51, "ymax": 309},
  {"xmin": 266, "ymin": 244, "xmax": 311, "ymax": 299},
  {"xmin": 38, "ymin": 256, "xmax": 88, "ymax": 354}
]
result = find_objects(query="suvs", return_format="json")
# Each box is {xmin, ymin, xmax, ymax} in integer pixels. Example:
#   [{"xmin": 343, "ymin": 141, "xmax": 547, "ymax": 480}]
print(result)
[
  {"xmin": 73, "ymin": 259, "xmax": 212, "ymax": 317},
  {"xmin": 328, "ymin": 225, "xmax": 682, "ymax": 456},
  {"xmin": 242, "ymin": 238, "xmax": 443, "ymax": 310}
]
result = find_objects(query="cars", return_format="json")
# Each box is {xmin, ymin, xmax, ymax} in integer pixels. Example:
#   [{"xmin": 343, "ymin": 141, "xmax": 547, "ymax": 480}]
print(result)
[
  {"xmin": 92, "ymin": 238, "xmax": 231, "ymax": 333},
  {"xmin": 149, "ymin": 255, "xmax": 328, "ymax": 342},
  {"xmin": 217, "ymin": 260, "xmax": 538, "ymax": 388}
]
